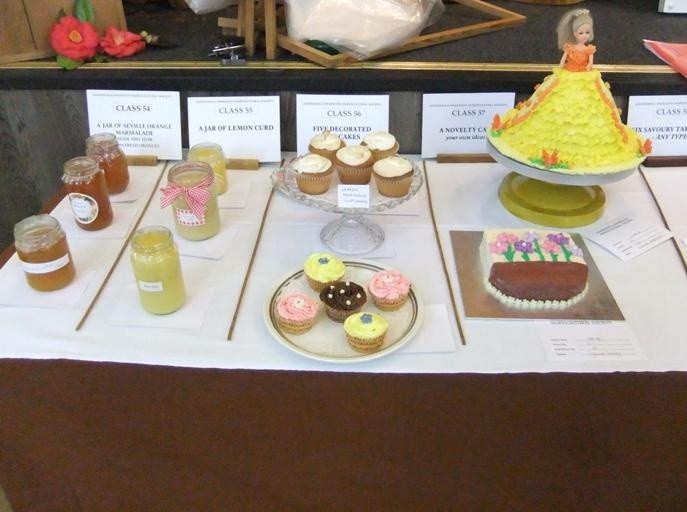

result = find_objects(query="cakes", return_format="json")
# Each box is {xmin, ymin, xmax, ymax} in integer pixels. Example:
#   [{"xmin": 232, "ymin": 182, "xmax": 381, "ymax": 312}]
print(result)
[
  {"xmin": 478, "ymin": 227, "xmax": 590, "ymax": 311},
  {"xmin": 485, "ymin": 8, "xmax": 654, "ymax": 170},
  {"xmin": 335, "ymin": 142, "xmax": 374, "ymax": 185},
  {"xmin": 360, "ymin": 130, "xmax": 401, "ymax": 161},
  {"xmin": 367, "ymin": 268, "xmax": 412, "ymax": 312},
  {"xmin": 343, "ymin": 311, "xmax": 389, "ymax": 355},
  {"xmin": 308, "ymin": 129, "xmax": 345, "ymax": 162},
  {"xmin": 302, "ymin": 251, "xmax": 347, "ymax": 292},
  {"xmin": 272, "ymin": 291, "xmax": 319, "ymax": 335},
  {"xmin": 371, "ymin": 154, "xmax": 415, "ymax": 198},
  {"xmin": 318, "ymin": 280, "xmax": 366, "ymax": 324},
  {"xmin": 289, "ymin": 153, "xmax": 335, "ymax": 197}
]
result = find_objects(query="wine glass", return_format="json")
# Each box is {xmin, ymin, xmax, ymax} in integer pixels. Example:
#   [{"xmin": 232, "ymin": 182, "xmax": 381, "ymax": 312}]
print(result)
[{"xmin": 271, "ymin": 154, "xmax": 423, "ymax": 256}]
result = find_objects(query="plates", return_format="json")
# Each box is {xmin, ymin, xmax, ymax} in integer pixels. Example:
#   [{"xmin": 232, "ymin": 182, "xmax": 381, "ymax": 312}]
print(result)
[{"xmin": 265, "ymin": 260, "xmax": 424, "ymax": 362}]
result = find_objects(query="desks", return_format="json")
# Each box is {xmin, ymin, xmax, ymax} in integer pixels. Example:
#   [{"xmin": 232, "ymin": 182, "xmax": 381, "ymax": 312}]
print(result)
[{"xmin": 0, "ymin": 152, "xmax": 684, "ymax": 511}]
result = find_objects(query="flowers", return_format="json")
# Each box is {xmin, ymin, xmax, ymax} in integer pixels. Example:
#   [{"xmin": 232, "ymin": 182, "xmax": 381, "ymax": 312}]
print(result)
[{"xmin": 49, "ymin": 1, "xmax": 177, "ymax": 72}]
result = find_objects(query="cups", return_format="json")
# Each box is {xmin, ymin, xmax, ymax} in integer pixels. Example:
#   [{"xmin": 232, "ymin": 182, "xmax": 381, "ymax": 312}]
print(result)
[
  {"xmin": 273, "ymin": 268, "xmax": 413, "ymax": 352},
  {"xmin": 290, "ymin": 140, "xmax": 416, "ymax": 197}
]
